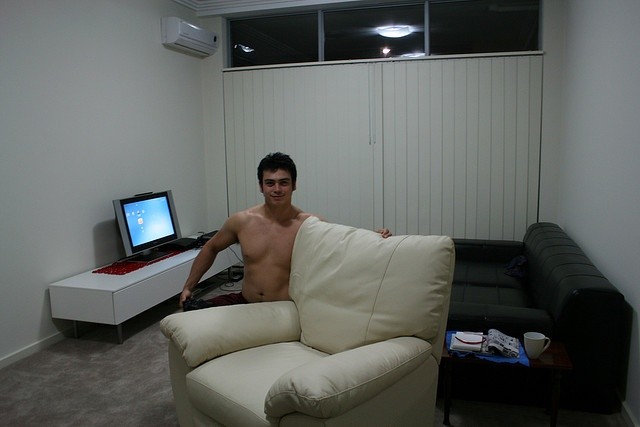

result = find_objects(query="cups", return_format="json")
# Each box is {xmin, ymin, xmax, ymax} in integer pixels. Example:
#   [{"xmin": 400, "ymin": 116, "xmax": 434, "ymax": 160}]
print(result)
[{"xmin": 523, "ymin": 332, "xmax": 550, "ymax": 359}]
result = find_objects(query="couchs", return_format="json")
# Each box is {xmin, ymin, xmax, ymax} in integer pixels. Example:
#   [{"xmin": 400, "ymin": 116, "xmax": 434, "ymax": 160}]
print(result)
[
  {"xmin": 397, "ymin": 221, "xmax": 625, "ymax": 427},
  {"xmin": 157, "ymin": 215, "xmax": 456, "ymax": 426}
]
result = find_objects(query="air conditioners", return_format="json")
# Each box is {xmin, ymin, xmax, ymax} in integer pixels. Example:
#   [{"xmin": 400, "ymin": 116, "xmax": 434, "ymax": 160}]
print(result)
[{"xmin": 161, "ymin": 16, "xmax": 218, "ymax": 58}]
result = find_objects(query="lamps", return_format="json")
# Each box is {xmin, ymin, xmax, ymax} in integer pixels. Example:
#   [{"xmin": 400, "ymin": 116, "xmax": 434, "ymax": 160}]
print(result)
[{"xmin": 372, "ymin": 23, "xmax": 425, "ymax": 42}]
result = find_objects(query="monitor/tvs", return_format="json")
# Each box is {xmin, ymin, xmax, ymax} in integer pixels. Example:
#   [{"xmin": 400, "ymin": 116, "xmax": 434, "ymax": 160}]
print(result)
[{"xmin": 112, "ymin": 190, "xmax": 182, "ymax": 263}]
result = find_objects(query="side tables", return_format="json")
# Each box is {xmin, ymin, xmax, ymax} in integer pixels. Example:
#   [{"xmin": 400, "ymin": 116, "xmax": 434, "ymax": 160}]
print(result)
[{"xmin": 440, "ymin": 335, "xmax": 574, "ymax": 427}]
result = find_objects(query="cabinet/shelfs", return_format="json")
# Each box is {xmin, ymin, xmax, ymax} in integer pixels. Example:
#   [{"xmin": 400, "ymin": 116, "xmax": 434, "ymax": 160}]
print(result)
[{"xmin": 47, "ymin": 228, "xmax": 243, "ymax": 344}]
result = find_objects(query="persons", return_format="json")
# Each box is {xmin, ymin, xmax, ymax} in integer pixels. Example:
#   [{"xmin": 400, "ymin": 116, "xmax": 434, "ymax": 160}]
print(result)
[{"xmin": 179, "ymin": 151, "xmax": 393, "ymax": 309}]
